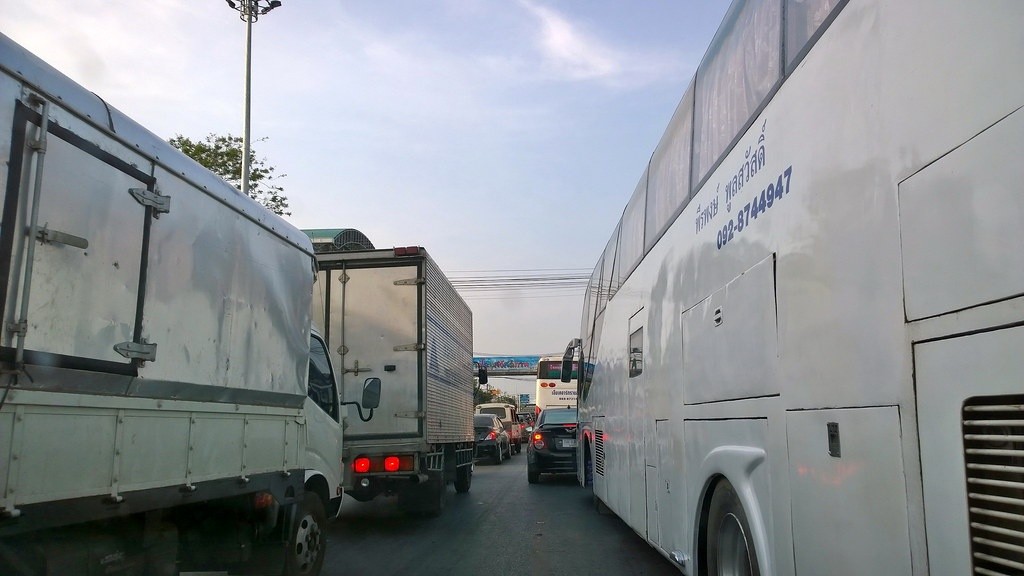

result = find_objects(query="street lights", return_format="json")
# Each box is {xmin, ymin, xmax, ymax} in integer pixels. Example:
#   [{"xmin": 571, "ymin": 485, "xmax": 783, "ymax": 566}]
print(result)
[{"xmin": 227, "ymin": 0, "xmax": 283, "ymax": 193}]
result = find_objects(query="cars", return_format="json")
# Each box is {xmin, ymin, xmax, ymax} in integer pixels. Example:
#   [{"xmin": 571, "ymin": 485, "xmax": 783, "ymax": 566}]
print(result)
[
  {"xmin": 526, "ymin": 407, "xmax": 580, "ymax": 484},
  {"xmin": 473, "ymin": 412, "xmax": 512, "ymax": 464}
]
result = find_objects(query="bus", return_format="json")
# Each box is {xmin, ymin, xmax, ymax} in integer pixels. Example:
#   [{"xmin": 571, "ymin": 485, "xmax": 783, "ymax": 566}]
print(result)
[
  {"xmin": 560, "ymin": 0, "xmax": 1022, "ymax": 574},
  {"xmin": 534, "ymin": 355, "xmax": 580, "ymax": 415}
]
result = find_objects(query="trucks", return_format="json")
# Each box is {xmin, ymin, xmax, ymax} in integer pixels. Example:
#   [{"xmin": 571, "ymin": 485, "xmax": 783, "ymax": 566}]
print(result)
[{"xmin": 304, "ymin": 242, "xmax": 489, "ymax": 521}]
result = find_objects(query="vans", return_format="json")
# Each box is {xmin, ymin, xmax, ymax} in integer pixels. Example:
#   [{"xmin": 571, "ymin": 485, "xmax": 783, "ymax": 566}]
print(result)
[{"xmin": 473, "ymin": 403, "xmax": 535, "ymax": 456}]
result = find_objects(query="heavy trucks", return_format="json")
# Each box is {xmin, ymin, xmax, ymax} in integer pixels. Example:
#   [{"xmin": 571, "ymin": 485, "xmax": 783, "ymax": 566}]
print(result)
[{"xmin": 0, "ymin": 29, "xmax": 383, "ymax": 576}]
